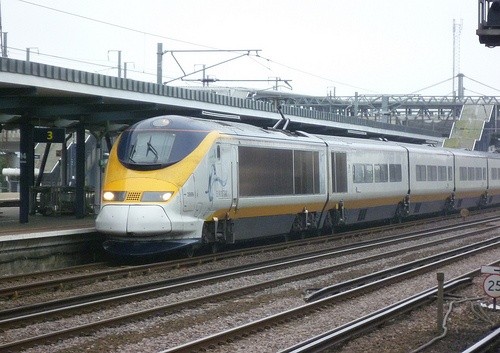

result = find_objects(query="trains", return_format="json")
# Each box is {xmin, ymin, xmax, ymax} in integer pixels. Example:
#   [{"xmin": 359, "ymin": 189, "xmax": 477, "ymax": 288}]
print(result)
[{"xmin": 95, "ymin": 114, "xmax": 499, "ymax": 258}]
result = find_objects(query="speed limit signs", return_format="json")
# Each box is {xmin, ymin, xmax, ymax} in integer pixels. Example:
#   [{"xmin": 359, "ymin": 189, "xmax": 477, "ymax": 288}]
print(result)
[{"xmin": 483, "ymin": 273, "xmax": 500, "ymax": 298}]
